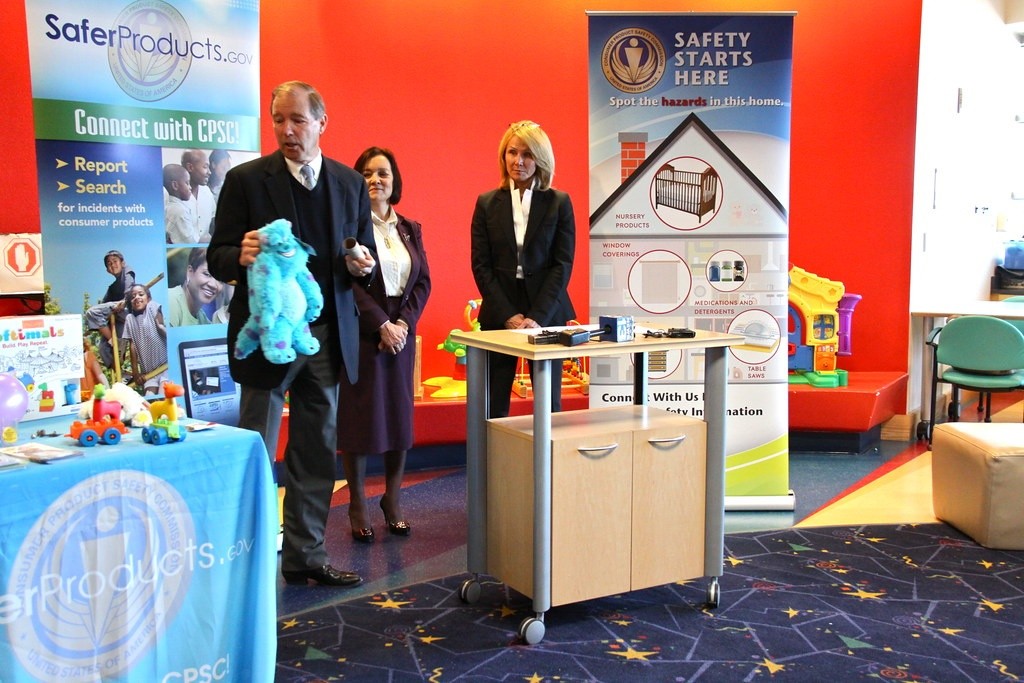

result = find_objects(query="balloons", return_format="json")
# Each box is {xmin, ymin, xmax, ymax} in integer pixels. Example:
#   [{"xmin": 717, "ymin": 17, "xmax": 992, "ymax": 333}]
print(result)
[{"xmin": 0, "ymin": 373, "xmax": 29, "ymax": 427}]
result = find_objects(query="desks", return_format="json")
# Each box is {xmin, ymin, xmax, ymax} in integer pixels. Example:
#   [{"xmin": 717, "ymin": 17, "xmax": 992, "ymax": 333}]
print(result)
[
  {"xmin": 911, "ymin": 301, "xmax": 1024, "ymax": 420},
  {"xmin": 0, "ymin": 399, "xmax": 276, "ymax": 683}
]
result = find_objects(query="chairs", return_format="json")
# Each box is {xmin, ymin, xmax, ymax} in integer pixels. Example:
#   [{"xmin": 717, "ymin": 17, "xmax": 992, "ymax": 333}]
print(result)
[{"xmin": 930, "ymin": 294, "xmax": 1024, "ymax": 439}]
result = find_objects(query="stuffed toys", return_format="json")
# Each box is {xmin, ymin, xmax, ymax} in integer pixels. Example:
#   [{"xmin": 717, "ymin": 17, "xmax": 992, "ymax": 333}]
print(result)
[
  {"xmin": 234, "ymin": 217, "xmax": 323, "ymax": 362},
  {"xmin": 78, "ymin": 382, "xmax": 146, "ymax": 425}
]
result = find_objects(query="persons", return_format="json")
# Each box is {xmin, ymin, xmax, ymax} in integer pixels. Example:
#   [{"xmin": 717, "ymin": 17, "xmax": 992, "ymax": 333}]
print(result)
[
  {"xmin": 204, "ymin": 80, "xmax": 364, "ymax": 590},
  {"xmin": 337, "ymin": 148, "xmax": 432, "ymax": 543},
  {"xmin": 471, "ymin": 120, "xmax": 575, "ymax": 418},
  {"xmin": 84, "ymin": 148, "xmax": 235, "ymax": 396}
]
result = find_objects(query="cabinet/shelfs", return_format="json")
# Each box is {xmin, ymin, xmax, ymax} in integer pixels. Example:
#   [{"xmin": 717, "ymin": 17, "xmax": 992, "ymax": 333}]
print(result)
[{"xmin": 447, "ymin": 323, "xmax": 747, "ymax": 641}]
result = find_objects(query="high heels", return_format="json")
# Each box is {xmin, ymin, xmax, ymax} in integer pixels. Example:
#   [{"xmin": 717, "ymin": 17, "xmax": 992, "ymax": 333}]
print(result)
[
  {"xmin": 380, "ymin": 493, "xmax": 410, "ymax": 536},
  {"xmin": 348, "ymin": 506, "xmax": 375, "ymax": 542}
]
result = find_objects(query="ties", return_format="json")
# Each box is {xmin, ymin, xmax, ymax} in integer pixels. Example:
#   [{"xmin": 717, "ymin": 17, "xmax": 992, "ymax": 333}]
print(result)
[{"xmin": 301, "ymin": 166, "xmax": 317, "ymax": 190}]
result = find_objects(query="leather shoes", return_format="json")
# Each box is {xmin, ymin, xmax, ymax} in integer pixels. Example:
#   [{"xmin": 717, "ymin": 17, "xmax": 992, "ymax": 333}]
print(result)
[{"xmin": 282, "ymin": 565, "xmax": 364, "ymax": 588}]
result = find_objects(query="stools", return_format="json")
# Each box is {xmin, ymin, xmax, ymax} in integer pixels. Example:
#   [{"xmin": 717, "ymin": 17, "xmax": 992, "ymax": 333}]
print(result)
[{"xmin": 930, "ymin": 422, "xmax": 1024, "ymax": 550}]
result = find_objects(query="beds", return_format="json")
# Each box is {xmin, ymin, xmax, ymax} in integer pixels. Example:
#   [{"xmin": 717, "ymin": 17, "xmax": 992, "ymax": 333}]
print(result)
[{"xmin": 655, "ymin": 163, "xmax": 717, "ymax": 223}]
